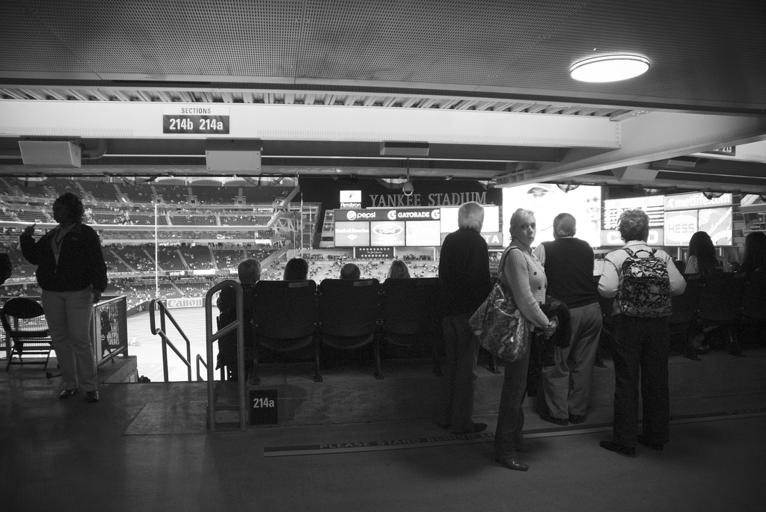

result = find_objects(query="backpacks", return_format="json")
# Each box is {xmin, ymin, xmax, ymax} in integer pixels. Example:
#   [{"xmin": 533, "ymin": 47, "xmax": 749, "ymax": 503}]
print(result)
[{"xmin": 616, "ymin": 248, "xmax": 674, "ymax": 321}]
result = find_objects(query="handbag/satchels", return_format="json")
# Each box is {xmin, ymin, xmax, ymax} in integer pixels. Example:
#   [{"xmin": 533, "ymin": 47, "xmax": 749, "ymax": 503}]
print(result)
[{"xmin": 467, "ymin": 279, "xmax": 531, "ymax": 364}]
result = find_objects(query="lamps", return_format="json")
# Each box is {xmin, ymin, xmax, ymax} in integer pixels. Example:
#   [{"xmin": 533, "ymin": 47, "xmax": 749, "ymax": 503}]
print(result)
[{"xmin": 568, "ymin": 51, "xmax": 650, "ymax": 82}]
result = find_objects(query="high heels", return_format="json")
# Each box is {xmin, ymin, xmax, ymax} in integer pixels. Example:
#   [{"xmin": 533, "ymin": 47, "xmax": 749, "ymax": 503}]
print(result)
[{"xmin": 494, "ymin": 430, "xmax": 531, "ymax": 471}]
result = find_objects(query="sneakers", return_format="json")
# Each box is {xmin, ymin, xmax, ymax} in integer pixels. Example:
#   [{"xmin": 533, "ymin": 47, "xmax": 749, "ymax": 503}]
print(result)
[
  {"xmin": 540, "ymin": 409, "xmax": 584, "ymax": 424},
  {"xmin": 635, "ymin": 434, "xmax": 663, "ymax": 451},
  {"xmin": 600, "ymin": 441, "xmax": 635, "ymax": 457}
]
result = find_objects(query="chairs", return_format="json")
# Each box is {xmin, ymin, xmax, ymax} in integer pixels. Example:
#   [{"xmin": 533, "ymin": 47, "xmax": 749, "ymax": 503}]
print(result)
[{"xmin": 0, "ymin": 297, "xmax": 56, "ymax": 371}]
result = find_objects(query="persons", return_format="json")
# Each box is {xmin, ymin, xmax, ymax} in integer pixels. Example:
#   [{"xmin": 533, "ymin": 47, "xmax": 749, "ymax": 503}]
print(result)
[
  {"xmin": 19, "ymin": 190, "xmax": 107, "ymax": 403},
  {"xmin": 477, "ymin": 207, "xmax": 561, "ymax": 474},
  {"xmin": 595, "ymin": 207, "xmax": 685, "ymax": 459},
  {"xmin": 437, "ymin": 202, "xmax": 490, "ymax": 438},
  {"xmin": 531, "ymin": 214, "xmax": 605, "ymax": 427},
  {"xmin": 238, "ymin": 257, "xmax": 410, "ymax": 284},
  {"xmin": 732, "ymin": 232, "xmax": 766, "ymax": 276},
  {"xmin": 683, "ymin": 230, "xmax": 725, "ymax": 275}
]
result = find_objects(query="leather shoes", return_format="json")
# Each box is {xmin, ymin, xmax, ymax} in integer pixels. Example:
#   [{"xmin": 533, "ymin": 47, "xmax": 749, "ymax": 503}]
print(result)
[
  {"xmin": 58, "ymin": 389, "xmax": 75, "ymax": 398},
  {"xmin": 87, "ymin": 392, "xmax": 99, "ymax": 401},
  {"xmin": 439, "ymin": 422, "xmax": 488, "ymax": 433}
]
